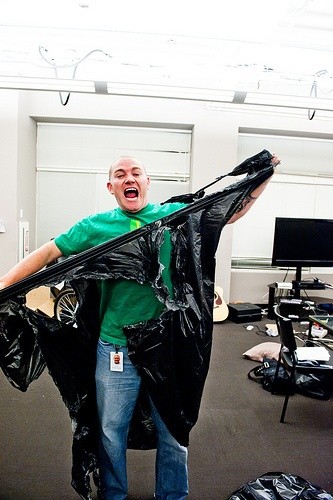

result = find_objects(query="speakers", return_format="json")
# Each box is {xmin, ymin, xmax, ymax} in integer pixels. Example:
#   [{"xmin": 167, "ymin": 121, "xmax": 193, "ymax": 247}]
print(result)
[{"xmin": 228, "ymin": 302, "xmax": 262, "ymax": 324}]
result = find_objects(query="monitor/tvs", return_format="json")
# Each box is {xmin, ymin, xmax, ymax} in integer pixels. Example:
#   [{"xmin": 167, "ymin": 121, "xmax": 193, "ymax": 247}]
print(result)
[{"xmin": 271, "ymin": 217, "xmax": 333, "ymax": 267}]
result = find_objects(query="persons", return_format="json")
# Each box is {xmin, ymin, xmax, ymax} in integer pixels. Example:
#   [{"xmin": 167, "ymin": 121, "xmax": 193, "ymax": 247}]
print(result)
[{"xmin": 1, "ymin": 153, "xmax": 284, "ymax": 500}]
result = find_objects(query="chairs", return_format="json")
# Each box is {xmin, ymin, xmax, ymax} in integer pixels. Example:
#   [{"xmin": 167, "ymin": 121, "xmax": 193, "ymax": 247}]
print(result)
[{"xmin": 269, "ymin": 302, "xmax": 333, "ymax": 423}]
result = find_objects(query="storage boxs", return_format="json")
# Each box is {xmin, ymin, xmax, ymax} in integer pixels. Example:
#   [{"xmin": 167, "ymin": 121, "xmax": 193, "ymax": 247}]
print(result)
[{"xmin": 227, "ymin": 301, "xmax": 263, "ymax": 325}]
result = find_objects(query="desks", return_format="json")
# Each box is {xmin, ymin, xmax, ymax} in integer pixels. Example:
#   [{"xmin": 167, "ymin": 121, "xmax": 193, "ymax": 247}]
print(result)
[{"xmin": 307, "ymin": 315, "xmax": 333, "ymax": 358}]
